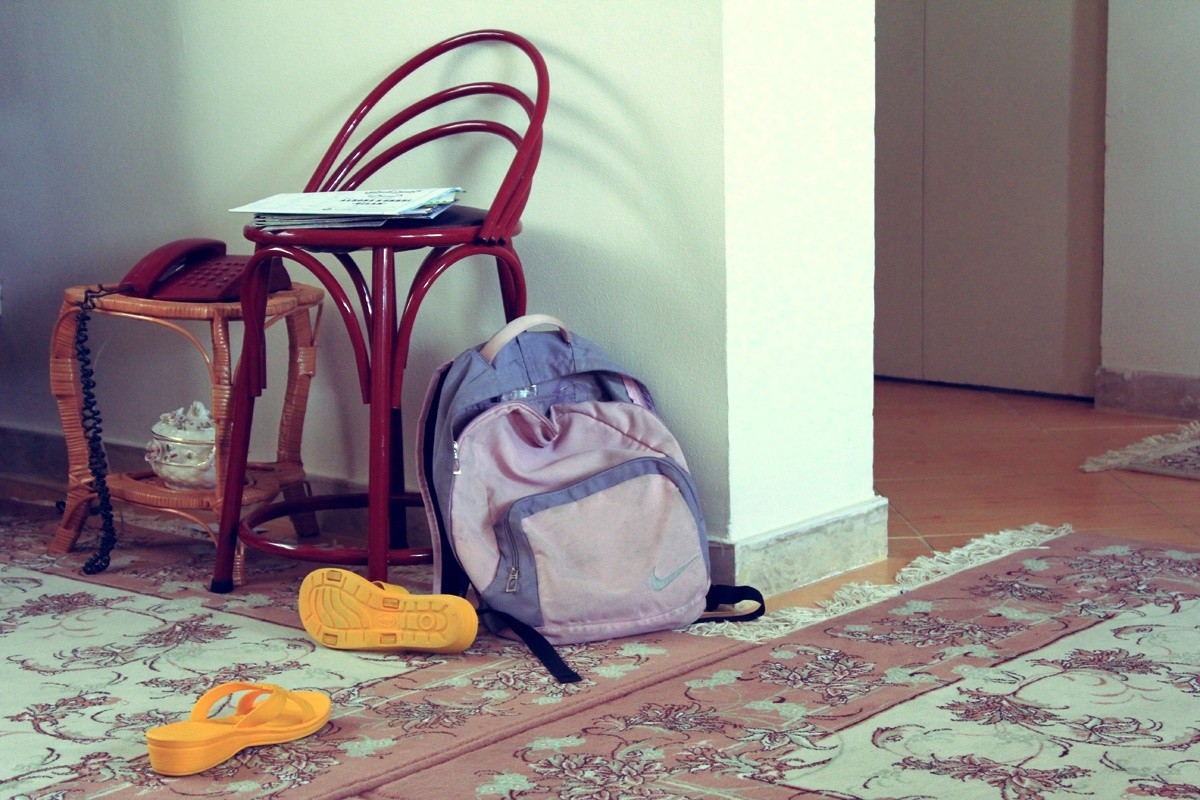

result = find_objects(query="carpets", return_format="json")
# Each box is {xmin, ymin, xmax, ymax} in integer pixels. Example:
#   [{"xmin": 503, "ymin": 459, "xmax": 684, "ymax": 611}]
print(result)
[
  {"xmin": 332, "ymin": 518, "xmax": 1200, "ymax": 800},
  {"xmin": 2, "ymin": 494, "xmax": 764, "ymax": 800},
  {"xmin": 1073, "ymin": 417, "xmax": 1200, "ymax": 485}
]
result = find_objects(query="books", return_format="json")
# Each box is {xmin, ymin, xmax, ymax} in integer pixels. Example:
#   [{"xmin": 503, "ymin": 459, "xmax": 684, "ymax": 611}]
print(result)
[{"xmin": 251, "ymin": 211, "xmax": 390, "ymax": 232}]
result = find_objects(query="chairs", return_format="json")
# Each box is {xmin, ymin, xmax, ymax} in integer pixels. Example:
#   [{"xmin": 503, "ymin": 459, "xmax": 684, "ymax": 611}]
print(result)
[{"xmin": 203, "ymin": 28, "xmax": 554, "ymax": 595}]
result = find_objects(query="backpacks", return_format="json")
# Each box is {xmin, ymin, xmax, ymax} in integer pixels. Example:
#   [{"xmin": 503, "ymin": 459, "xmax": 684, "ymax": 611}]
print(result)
[{"xmin": 415, "ymin": 313, "xmax": 765, "ymax": 684}]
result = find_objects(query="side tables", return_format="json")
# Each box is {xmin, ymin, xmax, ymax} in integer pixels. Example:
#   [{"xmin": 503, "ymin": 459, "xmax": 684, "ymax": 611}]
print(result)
[{"xmin": 49, "ymin": 277, "xmax": 329, "ymax": 586}]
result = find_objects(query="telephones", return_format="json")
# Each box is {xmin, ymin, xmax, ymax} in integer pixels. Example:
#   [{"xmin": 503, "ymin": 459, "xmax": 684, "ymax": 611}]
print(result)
[{"xmin": 123, "ymin": 237, "xmax": 295, "ymax": 307}]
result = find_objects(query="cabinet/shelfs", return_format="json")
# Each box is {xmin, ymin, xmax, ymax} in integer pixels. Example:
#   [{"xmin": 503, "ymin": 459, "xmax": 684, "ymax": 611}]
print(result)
[{"xmin": 876, "ymin": 0, "xmax": 1106, "ymax": 405}]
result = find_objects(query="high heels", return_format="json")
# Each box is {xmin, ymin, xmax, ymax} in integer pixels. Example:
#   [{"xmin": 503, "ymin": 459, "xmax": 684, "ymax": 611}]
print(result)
[
  {"xmin": 297, "ymin": 568, "xmax": 478, "ymax": 655},
  {"xmin": 144, "ymin": 683, "xmax": 331, "ymax": 777}
]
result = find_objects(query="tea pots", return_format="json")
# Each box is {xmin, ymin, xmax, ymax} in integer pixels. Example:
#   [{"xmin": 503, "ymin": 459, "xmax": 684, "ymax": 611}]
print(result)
[{"xmin": 147, "ymin": 401, "xmax": 218, "ymax": 491}]
